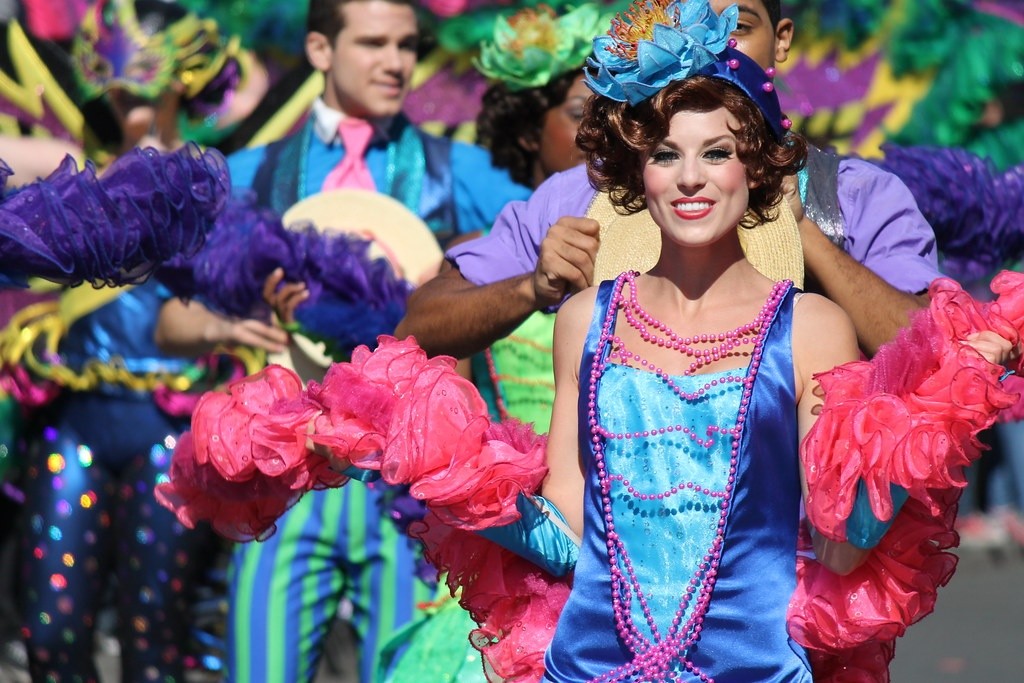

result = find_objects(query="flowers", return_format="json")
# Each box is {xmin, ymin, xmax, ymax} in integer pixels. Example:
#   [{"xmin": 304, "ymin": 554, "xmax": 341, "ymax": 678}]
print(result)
[{"xmin": 421, "ymin": 0, "xmax": 740, "ymax": 106}]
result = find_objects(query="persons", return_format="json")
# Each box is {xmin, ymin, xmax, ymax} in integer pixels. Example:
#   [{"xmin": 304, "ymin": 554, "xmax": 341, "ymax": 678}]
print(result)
[
  {"xmin": 89, "ymin": 0, "xmax": 537, "ymax": 682},
  {"xmin": 305, "ymin": 49, "xmax": 1017, "ymax": 683},
  {"xmin": 0, "ymin": 0, "xmax": 218, "ymax": 683},
  {"xmin": 377, "ymin": 65, "xmax": 602, "ymax": 682},
  {"xmin": 394, "ymin": 1, "xmax": 943, "ymax": 352}
]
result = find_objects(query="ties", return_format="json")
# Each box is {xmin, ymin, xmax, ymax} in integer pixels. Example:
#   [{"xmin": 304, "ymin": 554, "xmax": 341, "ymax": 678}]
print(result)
[{"xmin": 327, "ymin": 117, "xmax": 376, "ymax": 189}]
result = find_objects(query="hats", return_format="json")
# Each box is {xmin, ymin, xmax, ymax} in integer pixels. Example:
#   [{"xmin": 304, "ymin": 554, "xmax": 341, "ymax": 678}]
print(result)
[
  {"xmin": 576, "ymin": 184, "xmax": 805, "ymax": 296},
  {"xmin": 265, "ymin": 189, "xmax": 445, "ymax": 450}
]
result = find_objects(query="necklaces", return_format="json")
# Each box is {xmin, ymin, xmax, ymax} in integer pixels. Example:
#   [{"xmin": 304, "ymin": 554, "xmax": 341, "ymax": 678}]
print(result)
[{"xmin": 589, "ymin": 268, "xmax": 793, "ymax": 683}]
